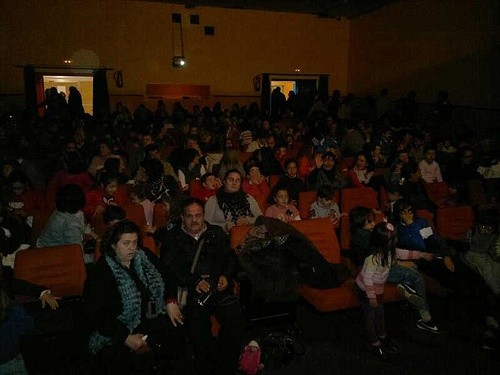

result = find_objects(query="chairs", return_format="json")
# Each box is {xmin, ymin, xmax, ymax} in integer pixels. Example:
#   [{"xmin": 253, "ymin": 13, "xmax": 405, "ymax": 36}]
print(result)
[{"xmin": 14, "ymin": 119, "xmax": 486, "ymax": 341}]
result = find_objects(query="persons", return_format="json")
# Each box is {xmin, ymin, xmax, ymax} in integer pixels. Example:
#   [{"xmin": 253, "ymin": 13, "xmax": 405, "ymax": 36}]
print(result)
[
  {"xmin": 0, "ymin": 279, "xmax": 63, "ymax": 375},
  {"xmin": 0, "ymin": 87, "xmax": 500, "ymax": 269},
  {"xmin": 354, "ymin": 222, "xmax": 433, "ymax": 360},
  {"xmin": 80, "ymin": 222, "xmax": 196, "ymax": 375},
  {"xmin": 349, "ymin": 206, "xmax": 448, "ymax": 334},
  {"xmin": 158, "ymin": 198, "xmax": 252, "ymax": 357},
  {"xmin": 394, "ymin": 198, "xmax": 500, "ymax": 351}
]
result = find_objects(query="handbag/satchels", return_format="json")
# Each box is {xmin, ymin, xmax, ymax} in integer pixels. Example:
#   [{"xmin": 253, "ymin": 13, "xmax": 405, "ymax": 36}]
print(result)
[{"xmin": 177, "ymin": 286, "xmax": 188, "ymax": 309}]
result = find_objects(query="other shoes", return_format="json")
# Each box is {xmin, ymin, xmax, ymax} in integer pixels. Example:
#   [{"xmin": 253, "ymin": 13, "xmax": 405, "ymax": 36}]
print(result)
[
  {"xmin": 378, "ymin": 335, "xmax": 399, "ymax": 350},
  {"xmin": 413, "ymin": 319, "xmax": 446, "ymax": 334},
  {"xmin": 372, "ymin": 344, "xmax": 387, "ymax": 361},
  {"xmin": 397, "ymin": 283, "xmax": 428, "ymax": 309}
]
